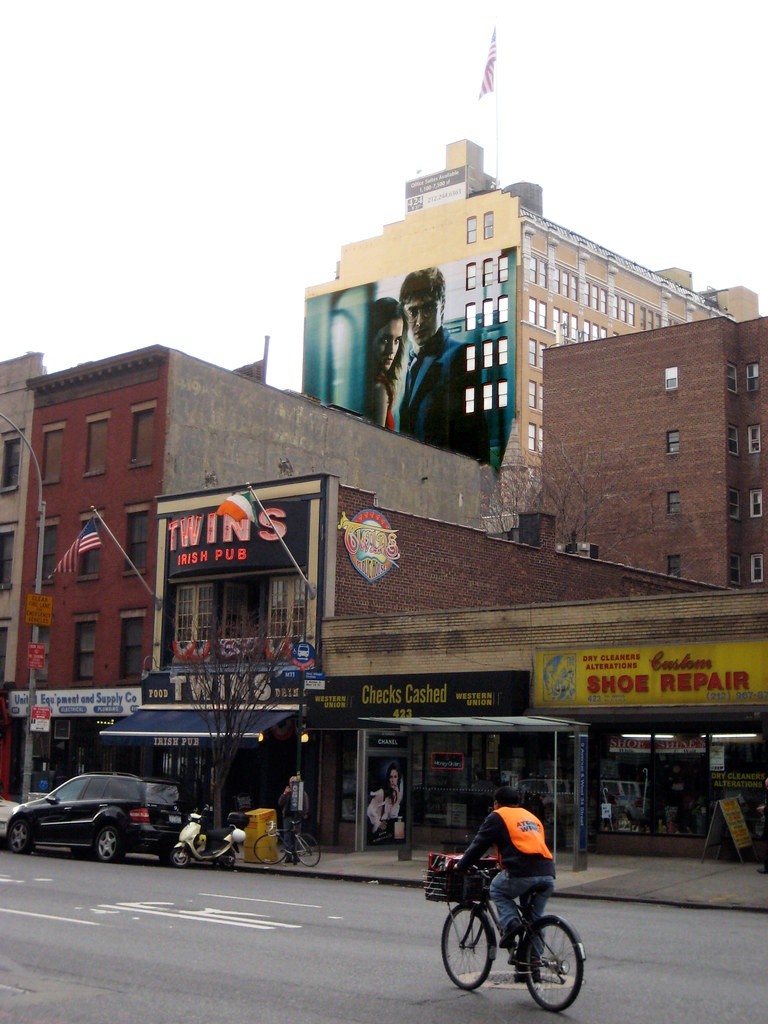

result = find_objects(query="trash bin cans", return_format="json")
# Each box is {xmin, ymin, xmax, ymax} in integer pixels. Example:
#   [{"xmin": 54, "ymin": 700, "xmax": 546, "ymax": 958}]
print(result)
[{"xmin": 243, "ymin": 807, "xmax": 278, "ymax": 863}]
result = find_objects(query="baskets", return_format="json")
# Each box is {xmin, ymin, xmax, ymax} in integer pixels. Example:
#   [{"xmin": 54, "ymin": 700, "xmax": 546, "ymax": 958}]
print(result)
[{"xmin": 421, "ymin": 866, "xmax": 501, "ymax": 902}]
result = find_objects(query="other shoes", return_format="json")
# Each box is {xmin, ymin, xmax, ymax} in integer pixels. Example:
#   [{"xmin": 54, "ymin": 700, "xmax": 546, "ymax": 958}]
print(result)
[
  {"xmin": 499, "ymin": 918, "xmax": 523, "ymax": 948},
  {"xmin": 514, "ymin": 969, "xmax": 540, "ymax": 983},
  {"xmin": 285, "ymin": 858, "xmax": 291, "ymax": 862}
]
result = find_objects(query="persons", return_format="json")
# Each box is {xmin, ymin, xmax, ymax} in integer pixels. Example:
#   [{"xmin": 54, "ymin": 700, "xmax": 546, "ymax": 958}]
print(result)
[
  {"xmin": 369, "ymin": 297, "xmax": 410, "ymax": 435},
  {"xmin": 453, "ymin": 785, "xmax": 560, "ymax": 973},
  {"xmin": 279, "ymin": 775, "xmax": 308, "ymax": 864},
  {"xmin": 366, "ymin": 763, "xmax": 404, "ymax": 834},
  {"xmin": 756, "ymin": 777, "xmax": 768, "ymax": 874},
  {"xmin": 399, "ymin": 266, "xmax": 466, "ymax": 455}
]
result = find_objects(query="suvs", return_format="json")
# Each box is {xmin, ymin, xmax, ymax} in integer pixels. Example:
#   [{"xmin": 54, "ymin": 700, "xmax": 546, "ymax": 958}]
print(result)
[
  {"xmin": 6, "ymin": 772, "xmax": 189, "ymax": 865},
  {"xmin": 518, "ymin": 778, "xmax": 650, "ymax": 828}
]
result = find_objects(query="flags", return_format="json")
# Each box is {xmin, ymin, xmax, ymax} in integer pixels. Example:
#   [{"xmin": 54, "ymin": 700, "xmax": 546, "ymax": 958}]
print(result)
[
  {"xmin": 51, "ymin": 517, "xmax": 105, "ymax": 575},
  {"xmin": 215, "ymin": 491, "xmax": 261, "ymax": 530},
  {"xmin": 475, "ymin": 23, "xmax": 498, "ymax": 104}
]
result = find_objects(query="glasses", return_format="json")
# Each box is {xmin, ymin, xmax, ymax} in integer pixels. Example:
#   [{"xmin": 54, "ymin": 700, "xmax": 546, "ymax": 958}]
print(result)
[{"xmin": 408, "ymin": 303, "xmax": 440, "ymax": 323}]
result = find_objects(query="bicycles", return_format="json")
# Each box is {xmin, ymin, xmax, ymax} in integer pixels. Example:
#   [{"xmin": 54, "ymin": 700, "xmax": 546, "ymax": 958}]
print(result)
[
  {"xmin": 422, "ymin": 865, "xmax": 585, "ymax": 1012},
  {"xmin": 254, "ymin": 819, "xmax": 321, "ymax": 868}
]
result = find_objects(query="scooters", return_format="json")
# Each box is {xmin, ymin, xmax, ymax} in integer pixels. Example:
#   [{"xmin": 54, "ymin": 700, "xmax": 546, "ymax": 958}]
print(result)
[{"xmin": 170, "ymin": 805, "xmax": 250, "ymax": 870}]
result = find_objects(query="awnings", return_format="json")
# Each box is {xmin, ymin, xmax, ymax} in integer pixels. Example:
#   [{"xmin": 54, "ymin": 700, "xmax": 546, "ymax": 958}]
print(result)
[
  {"xmin": 100, "ymin": 708, "xmax": 299, "ymax": 738},
  {"xmin": 357, "ymin": 712, "xmax": 593, "ymax": 729}
]
